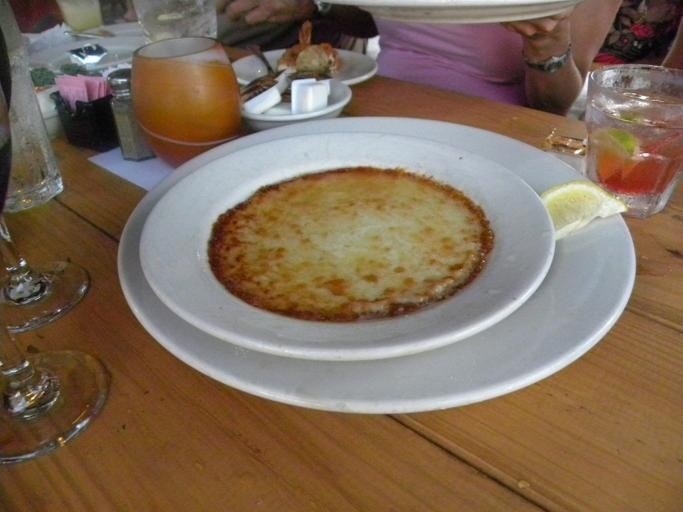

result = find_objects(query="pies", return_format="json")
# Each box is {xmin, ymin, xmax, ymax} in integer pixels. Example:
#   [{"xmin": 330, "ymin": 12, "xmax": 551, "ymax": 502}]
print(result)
[{"xmin": 209, "ymin": 168, "xmax": 495, "ymax": 323}]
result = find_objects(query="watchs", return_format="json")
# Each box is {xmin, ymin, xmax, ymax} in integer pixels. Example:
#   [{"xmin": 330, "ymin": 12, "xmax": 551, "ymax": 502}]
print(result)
[{"xmin": 522, "ymin": 42, "xmax": 572, "ymax": 76}]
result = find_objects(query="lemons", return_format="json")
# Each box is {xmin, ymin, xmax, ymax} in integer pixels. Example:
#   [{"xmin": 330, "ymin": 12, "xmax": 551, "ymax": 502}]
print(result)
[
  {"xmin": 589, "ymin": 127, "xmax": 635, "ymax": 157},
  {"xmin": 541, "ymin": 181, "xmax": 629, "ymax": 236}
]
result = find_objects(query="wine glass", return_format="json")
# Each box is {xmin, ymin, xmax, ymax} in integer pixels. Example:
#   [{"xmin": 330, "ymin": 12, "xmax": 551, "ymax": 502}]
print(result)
[{"xmin": 0, "ymin": 97, "xmax": 106, "ymax": 464}]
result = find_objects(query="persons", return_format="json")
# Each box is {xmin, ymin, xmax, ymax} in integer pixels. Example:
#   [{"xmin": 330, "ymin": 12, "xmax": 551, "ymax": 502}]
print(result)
[{"xmin": 213, "ymin": 1, "xmax": 625, "ymax": 117}]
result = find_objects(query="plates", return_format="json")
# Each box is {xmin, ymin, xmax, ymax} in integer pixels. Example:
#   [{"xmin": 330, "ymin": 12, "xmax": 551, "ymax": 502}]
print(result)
[
  {"xmin": 117, "ymin": 114, "xmax": 636, "ymax": 416},
  {"xmin": 325, "ymin": 1, "xmax": 586, "ymax": 24},
  {"xmin": 139, "ymin": 128, "xmax": 554, "ymax": 363},
  {"xmin": 49, "ymin": 1, "xmax": 381, "ymax": 170}
]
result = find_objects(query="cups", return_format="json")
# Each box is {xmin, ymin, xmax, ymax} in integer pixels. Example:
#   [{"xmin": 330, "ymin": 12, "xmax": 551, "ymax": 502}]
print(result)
[
  {"xmin": 583, "ymin": 61, "xmax": 681, "ymax": 216},
  {"xmin": 0, "ymin": 2, "xmax": 66, "ymax": 213}
]
